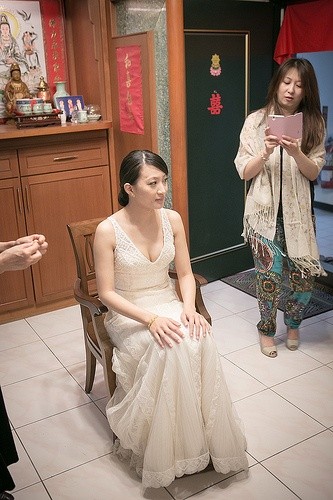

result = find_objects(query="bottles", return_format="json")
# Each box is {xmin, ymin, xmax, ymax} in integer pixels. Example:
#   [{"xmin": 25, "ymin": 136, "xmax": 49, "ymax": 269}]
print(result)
[{"xmin": 52, "ymin": 81, "xmax": 70, "ymax": 109}]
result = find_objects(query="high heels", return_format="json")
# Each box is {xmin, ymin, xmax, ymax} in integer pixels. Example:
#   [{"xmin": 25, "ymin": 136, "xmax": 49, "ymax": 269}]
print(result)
[
  {"xmin": 286, "ymin": 327, "xmax": 300, "ymax": 352},
  {"xmin": 258, "ymin": 331, "xmax": 277, "ymax": 358}
]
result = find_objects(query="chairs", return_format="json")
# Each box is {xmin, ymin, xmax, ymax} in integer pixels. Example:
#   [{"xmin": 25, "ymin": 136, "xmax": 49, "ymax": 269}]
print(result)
[{"xmin": 67, "ymin": 218, "xmax": 212, "ymax": 397}]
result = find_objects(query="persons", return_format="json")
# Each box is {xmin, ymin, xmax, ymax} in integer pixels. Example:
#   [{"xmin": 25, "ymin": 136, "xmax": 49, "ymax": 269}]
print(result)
[
  {"xmin": 0, "ymin": 234, "xmax": 47, "ymax": 500},
  {"xmin": 234, "ymin": 58, "xmax": 328, "ymax": 357},
  {"xmin": 4, "ymin": 63, "xmax": 30, "ymax": 116},
  {"xmin": 94, "ymin": 149, "xmax": 248, "ymax": 489}
]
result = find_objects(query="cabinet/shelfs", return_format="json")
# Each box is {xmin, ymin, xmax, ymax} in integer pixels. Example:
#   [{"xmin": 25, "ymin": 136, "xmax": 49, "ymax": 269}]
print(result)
[{"xmin": 0, "ymin": 125, "xmax": 112, "ymax": 320}]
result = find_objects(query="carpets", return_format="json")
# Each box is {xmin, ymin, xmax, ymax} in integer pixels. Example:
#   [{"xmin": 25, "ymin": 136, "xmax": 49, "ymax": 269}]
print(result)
[{"xmin": 221, "ymin": 269, "xmax": 333, "ymax": 318}]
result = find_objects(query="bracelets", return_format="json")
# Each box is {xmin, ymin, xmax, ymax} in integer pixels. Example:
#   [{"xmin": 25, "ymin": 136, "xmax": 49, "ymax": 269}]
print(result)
[
  {"xmin": 260, "ymin": 152, "xmax": 270, "ymax": 161},
  {"xmin": 148, "ymin": 315, "xmax": 158, "ymax": 330}
]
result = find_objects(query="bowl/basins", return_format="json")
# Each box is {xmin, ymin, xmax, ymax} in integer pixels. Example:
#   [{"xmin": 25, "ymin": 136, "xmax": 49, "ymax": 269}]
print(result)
[{"xmin": 87, "ymin": 115, "xmax": 101, "ymax": 121}]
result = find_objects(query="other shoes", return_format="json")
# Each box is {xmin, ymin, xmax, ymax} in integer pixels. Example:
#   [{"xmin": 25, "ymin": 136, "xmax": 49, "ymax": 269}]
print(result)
[{"xmin": 0, "ymin": 491, "xmax": 14, "ymax": 500}]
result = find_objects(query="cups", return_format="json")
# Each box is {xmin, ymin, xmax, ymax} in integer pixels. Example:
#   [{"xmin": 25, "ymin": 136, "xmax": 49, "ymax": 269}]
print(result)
[
  {"xmin": 15, "ymin": 98, "xmax": 53, "ymax": 115},
  {"xmin": 76, "ymin": 111, "xmax": 86, "ymax": 122}
]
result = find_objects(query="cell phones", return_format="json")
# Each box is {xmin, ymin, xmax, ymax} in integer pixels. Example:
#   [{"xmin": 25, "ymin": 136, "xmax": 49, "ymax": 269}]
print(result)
[{"xmin": 267, "ymin": 112, "xmax": 303, "ymax": 141}]
